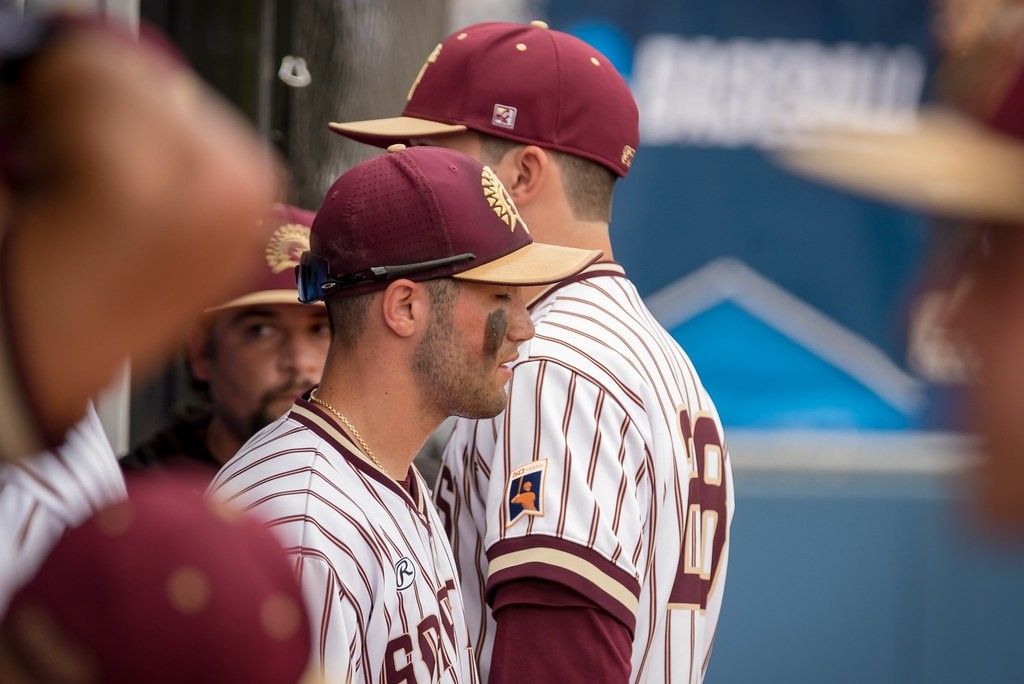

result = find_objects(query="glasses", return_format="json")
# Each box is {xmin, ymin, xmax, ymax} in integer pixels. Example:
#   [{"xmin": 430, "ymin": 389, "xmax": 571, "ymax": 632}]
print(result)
[{"xmin": 297, "ymin": 250, "xmax": 474, "ymax": 303}]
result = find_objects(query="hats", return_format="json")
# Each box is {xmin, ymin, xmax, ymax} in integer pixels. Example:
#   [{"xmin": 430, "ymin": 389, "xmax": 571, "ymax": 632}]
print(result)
[
  {"xmin": 328, "ymin": 16, "xmax": 641, "ymax": 175},
  {"xmin": 27, "ymin": 478, "xmax": 311, "ymax": 684},
  {"xmin": 202, "ymin": 204, "xmax": 319, "ymax": 315},
  {"xmin": 294, "ymin": 142, "xmax": 603, "ymax": 303},
  {"xmin": 772, "ymin": 0, "xmax": 1024, "ymax": 223}
]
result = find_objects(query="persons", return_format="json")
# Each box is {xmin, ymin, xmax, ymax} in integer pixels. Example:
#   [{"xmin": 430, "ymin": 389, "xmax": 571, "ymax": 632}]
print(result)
[
  {"xmin": 0, "ymin": 494, "xmax": 311, "ymax": 684},
  {"xmin": 327, "ymin": 20, "xmax": 733, "ymax": 684},
  {"xmin": 775, "ymin": 0, "xmax": 1024, "ymax": 535},
  {"xmin": 204, "ymin": 144, "xmax": 603, "ymax": 684},
  {"xmin": 0, "ymin": 12, "xmax": 277, "ymax": 606},
  {"xmin": 119, "ymin": 203, "xmax": 332, "ymax": 486}
]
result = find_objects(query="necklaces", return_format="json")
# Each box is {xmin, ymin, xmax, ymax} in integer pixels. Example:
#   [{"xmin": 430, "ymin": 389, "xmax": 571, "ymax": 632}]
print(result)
[{"xmin": 310, "ymin": 387, "xmax": 386, "ymax": 471}]
